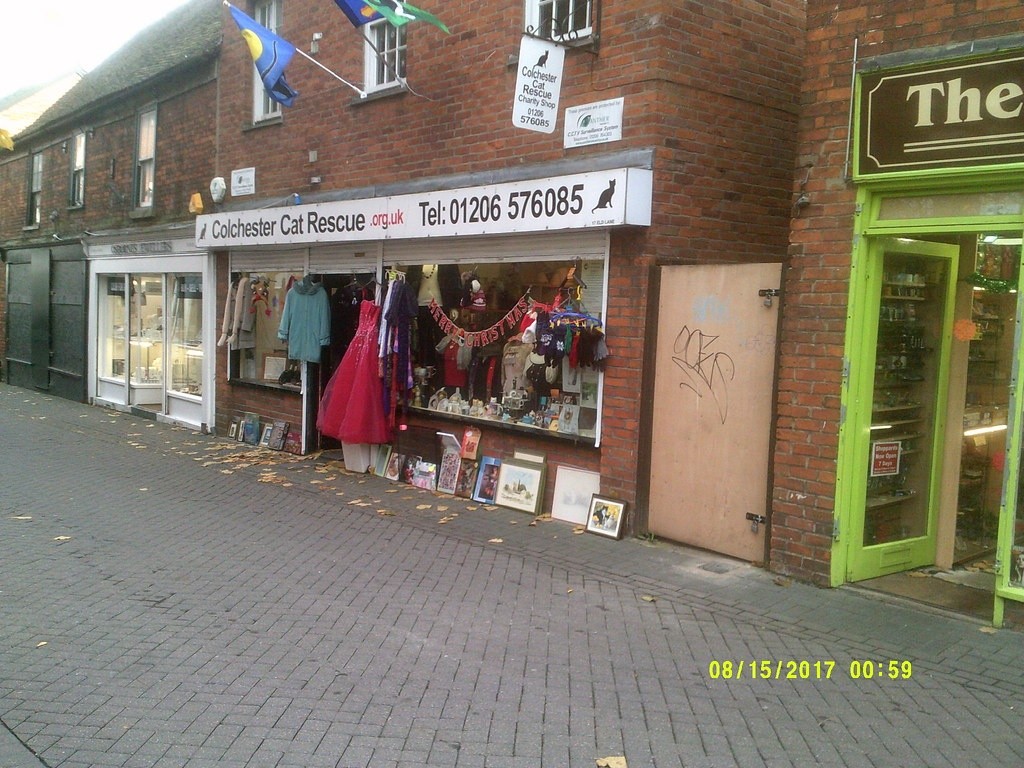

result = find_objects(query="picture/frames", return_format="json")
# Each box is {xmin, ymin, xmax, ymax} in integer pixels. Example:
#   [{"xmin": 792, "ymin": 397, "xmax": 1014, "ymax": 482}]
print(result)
[
  {"xmin": 512, "ymin": 448, "xmax": 547, "ymax": 464},
  {"xmin": 473, "ymin": 455, "xmax": 500, "ymax": 504},
  {"xmin": 503, "ymin": 456, "xmax": 547, "ymax": 515},
  {"xmin": 493, "ymin": 459, "xmax": 545, "ymax": 516},
  {"xmin": 261, "ymin": 352, "xmax": 289, "ymax": 383},
  {"xmin": 227, "ymin": 420, "xmax": 238, "ymax": 440},
  {"xmin": 373, "ymin": 444, "xmax": 392, "ymax": 477},
  {"xmin": 259, "ymin": 423, "xmax": 273, "ymax": 448},
  {"xmin": 584, "ymin": 494, "xmax": 628, "ymax": 541}
]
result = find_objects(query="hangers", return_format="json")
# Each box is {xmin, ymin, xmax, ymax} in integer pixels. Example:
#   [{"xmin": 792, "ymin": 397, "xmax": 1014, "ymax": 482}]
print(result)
[
  {"xmin": 298, "ymin": 269, "xmax": 315, "ymax": 286},
  {"xmin": 344, "ymin": 272, "xmax": 368, "ymax": 290},
  {"xmin": 357, "ymin": 273, "xmax": 386, "ymax": 290}
]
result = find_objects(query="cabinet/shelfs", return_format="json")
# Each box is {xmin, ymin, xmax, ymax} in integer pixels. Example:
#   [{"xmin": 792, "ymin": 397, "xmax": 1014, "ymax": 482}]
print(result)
[
  {"xmin": 959, "ymin": 277, "xmax": 1009, "ymax": 495},
  {"xmin": 866, "ymin": 281, "xmax": 934, "ymax": 512}
]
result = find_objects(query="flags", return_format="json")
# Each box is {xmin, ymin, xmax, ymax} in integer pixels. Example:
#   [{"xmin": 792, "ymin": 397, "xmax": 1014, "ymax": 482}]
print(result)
[
  {"xmin": 230, "ymin": 4, "xmax": 301, "ymax": 107},
  {"xmin": 336, "ymin": 0, "xmax": 453, "ymax": 40}
]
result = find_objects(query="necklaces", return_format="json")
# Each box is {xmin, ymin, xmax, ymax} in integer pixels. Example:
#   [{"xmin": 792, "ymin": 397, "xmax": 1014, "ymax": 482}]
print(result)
[{"xmin": 422, "ymin": 265, "xmax": 435, "ymax": 280}]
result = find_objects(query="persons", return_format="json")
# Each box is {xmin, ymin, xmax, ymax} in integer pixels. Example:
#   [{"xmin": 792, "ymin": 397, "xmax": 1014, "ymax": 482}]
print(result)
[
  {"xmin": 406, "ymin": 264, "xmax": 462, "ymax": 388},
  {"xmin": 523, "ymin": 339, "xmax": 558, "ymax": 402},
  {"xmin": 501, "ymin": 332, "xmax": 532, "ymax": 396}
]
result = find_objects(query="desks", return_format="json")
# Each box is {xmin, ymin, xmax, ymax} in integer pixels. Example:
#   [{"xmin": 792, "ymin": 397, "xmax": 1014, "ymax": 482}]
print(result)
[
  {"xmin": 112, "ymin": 335, "xmax": 161, "ymax": 378},
  {"xmin": 177, "ymin": 344, "xmax": 203, "ymax": 388}
]
